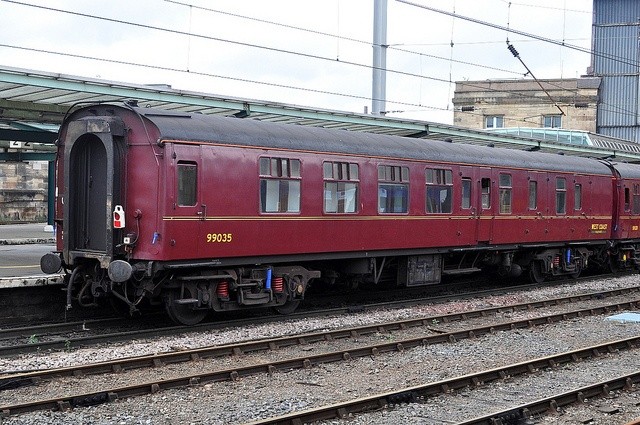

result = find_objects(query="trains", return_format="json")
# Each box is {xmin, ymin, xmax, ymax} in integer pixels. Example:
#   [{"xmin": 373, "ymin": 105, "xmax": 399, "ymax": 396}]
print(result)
[{"xmin": 40, "ymin": 97, "xmax": 640, "ymax": 326}]
